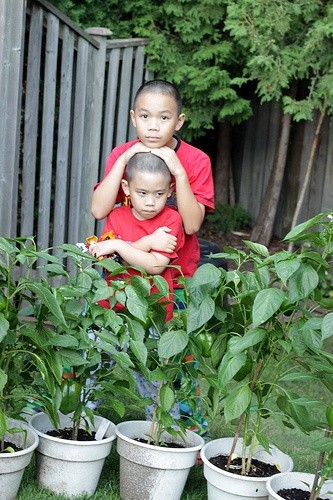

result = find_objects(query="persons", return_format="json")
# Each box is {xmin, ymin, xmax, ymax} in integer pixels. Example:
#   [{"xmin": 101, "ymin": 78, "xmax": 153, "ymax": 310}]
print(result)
[
  {"xmin": 50, "ymin": 79, "xmax": 216, "ymax": 437},
  {"xmin": 79, "ymin": 152, "xmax": 182, "ymax": 428}
]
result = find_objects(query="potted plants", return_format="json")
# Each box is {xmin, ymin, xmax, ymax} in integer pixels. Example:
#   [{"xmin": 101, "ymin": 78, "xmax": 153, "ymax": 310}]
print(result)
[{"xmin": 0, "ymin": 207, "xmax": 333, "ymax": 500}]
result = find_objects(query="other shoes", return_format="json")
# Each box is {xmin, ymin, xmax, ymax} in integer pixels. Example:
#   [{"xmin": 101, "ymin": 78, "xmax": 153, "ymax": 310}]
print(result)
[{"xmin": 183, "ymin": 415, "xmax": 209, "ymax": 435}]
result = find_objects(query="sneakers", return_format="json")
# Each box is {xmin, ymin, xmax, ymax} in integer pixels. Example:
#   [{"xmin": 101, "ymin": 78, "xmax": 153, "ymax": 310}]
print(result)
[{"xmin": 18, "ymin": 397, "xmax": 45, "ymax": 423}]
